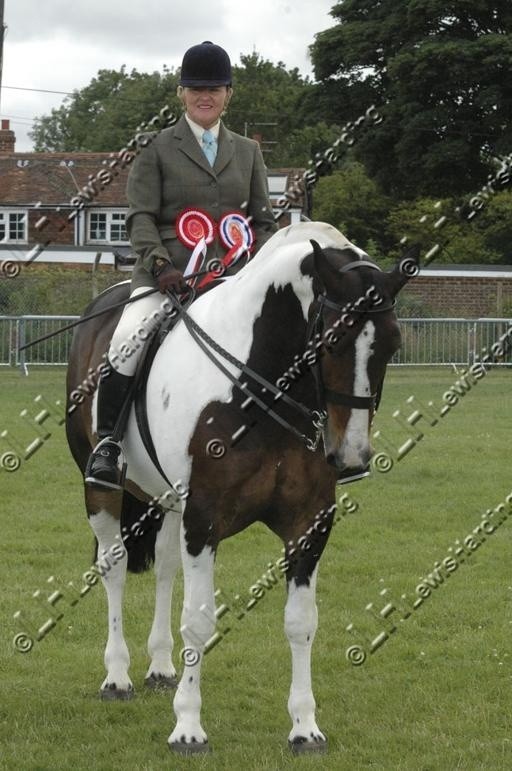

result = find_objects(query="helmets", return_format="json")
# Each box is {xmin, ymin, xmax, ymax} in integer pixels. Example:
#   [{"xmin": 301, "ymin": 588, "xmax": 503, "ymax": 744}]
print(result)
[{"xmin": 180, "ymin": 42, "xmax": 232, "ymax": 90}]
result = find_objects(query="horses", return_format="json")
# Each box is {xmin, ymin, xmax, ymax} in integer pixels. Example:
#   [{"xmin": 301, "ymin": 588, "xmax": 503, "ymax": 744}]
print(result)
[{"xmin": 66, "ymin": 221, "xmax": 422, "ymax": 756}]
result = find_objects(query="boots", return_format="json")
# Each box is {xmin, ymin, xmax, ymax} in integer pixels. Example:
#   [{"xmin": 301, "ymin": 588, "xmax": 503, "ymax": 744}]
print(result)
[{"xmin": 90, "ymin": 355, "xmax": 134, "ymax": 491}]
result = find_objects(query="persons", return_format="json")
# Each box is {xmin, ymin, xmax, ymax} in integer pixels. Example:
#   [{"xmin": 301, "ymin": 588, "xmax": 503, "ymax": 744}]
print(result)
[{"xmin": 85, "ymin": 41, "xmax": 371, "ymax": 489}]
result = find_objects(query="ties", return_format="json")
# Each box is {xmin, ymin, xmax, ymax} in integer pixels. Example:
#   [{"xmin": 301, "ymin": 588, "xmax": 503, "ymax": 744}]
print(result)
[{"xmin": 202, "ymin": 132, "xmax": 215, "ymax": 168}]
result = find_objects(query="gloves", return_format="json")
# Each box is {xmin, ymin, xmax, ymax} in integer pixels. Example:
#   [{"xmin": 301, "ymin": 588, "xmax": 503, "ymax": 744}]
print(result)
[{"xmin": 152, "ymin": 257, "xmax": 186, "ymax": 294}]
